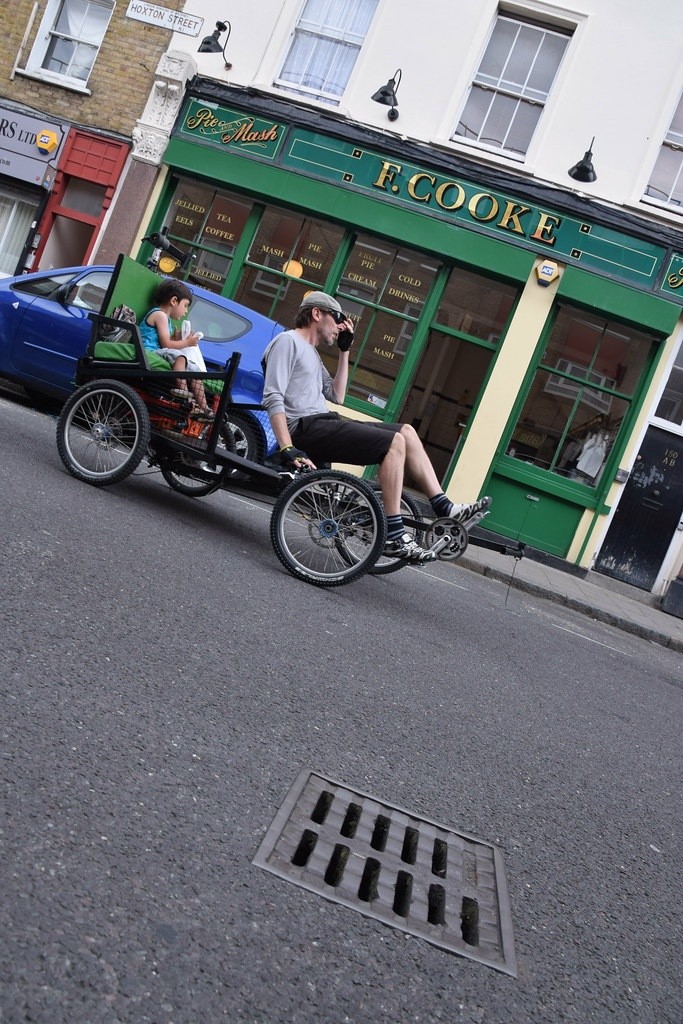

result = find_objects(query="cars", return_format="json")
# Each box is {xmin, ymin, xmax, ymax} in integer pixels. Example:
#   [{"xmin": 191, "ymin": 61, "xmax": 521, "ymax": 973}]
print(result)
[{"xmin": 0, "ymin": 260, "xmax": 337, "ymax": 492}]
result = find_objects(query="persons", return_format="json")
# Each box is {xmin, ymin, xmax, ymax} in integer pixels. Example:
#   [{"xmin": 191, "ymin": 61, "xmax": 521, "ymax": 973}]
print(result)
[
  {"xmin": 262, "ymin": 291, "xmax": 492, "ymax": 562},
  {"xmin": 139, "ymin": 279, "xmax": 216, "ymax": 419}
]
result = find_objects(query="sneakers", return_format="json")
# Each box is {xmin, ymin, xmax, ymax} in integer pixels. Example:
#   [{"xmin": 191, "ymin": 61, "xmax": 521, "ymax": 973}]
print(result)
[
  {"xmin": 383, "ymin": 533, "xmax": 440, "ymax": 563},
  {"xmin": 448, "ymin": 496, "xmax": 492, "ymax": 524}
]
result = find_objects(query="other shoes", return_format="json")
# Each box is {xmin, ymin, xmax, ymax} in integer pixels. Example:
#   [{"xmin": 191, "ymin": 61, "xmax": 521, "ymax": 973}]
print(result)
[{"xmin": 190, "ymin": 408, "xmax": 216, "ymax": 420}]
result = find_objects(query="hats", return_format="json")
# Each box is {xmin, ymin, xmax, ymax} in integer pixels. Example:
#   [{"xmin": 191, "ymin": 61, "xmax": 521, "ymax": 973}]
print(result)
[{"xmin": 300, "ymin": 291, "xmax": 347, "ymax": 321}]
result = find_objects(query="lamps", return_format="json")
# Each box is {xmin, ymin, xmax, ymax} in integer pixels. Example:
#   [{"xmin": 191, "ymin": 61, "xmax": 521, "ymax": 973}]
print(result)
[
  {"xmin": 197, "ymin": 20, "xmax": 232, "ymax": 71},
  {"xmin": 159, "ymin": 217, "xmax": 193, "ymax": 274},
  {"xmin": 371, "ymin": 67, "xmax": 402, "ymax": 121},
  {"xmin": 304, "ymin": 249, "xmax": 329, "ymax": 298},
  {"xmin": 568, "ymin": 136, "xmax": 597, "ymax": 183},
  {"xmin": 283, "ymin": 219, "xmax": 313, "ymax": 279}
]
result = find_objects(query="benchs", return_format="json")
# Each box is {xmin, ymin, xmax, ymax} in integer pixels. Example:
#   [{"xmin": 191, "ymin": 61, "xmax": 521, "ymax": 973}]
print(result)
[{"xmin": 87, "ymin": 253, "xmax": 225, "ymax": 406}]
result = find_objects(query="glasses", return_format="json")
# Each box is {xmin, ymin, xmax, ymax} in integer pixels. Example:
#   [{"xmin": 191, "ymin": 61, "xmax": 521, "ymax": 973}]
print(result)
[{"xmin": 321, "ymin": 308, "xmax": 344, "ymax": 324}]
